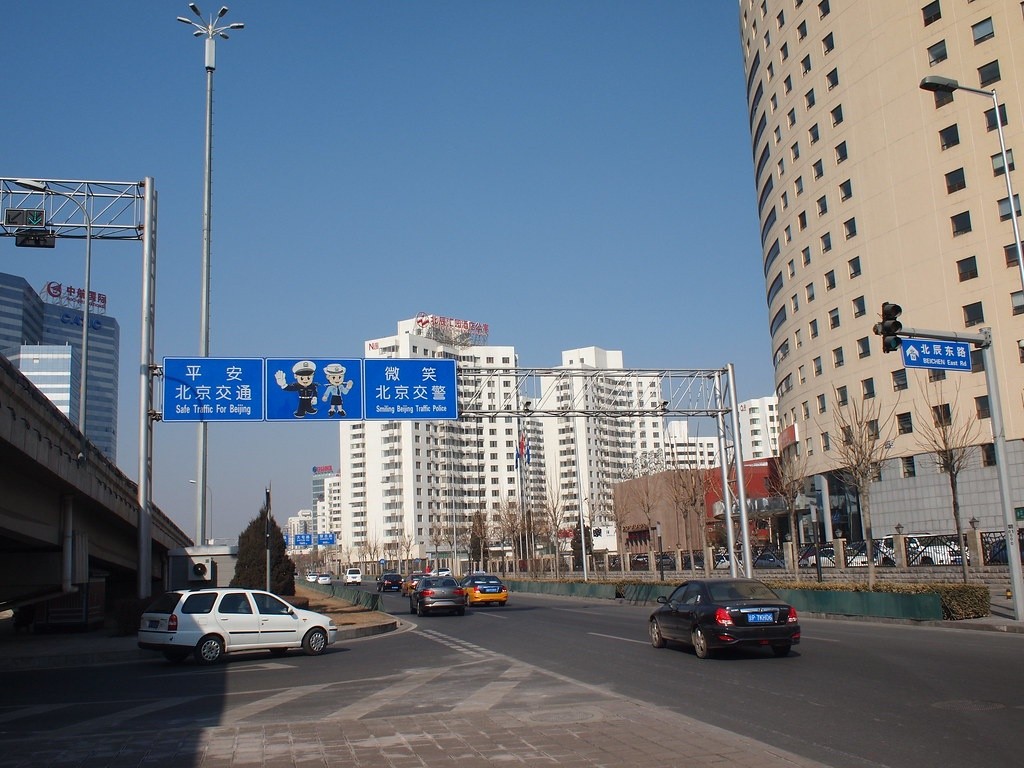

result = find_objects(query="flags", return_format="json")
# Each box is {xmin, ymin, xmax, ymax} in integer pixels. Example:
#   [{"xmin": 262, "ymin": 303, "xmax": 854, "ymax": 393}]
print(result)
[
  {"xmin": 519, "ymin": 435, "xmax": 524, "ymax": 456},
  {"xmin": 515, "ymin": 446, "xmax": 518, "ymax": 469},
  {"xmin": 525, "ymin": 439, "xmax": 530, "ymax": 464}
]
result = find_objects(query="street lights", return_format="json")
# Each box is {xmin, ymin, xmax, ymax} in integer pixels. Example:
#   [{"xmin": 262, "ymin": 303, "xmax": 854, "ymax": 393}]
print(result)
[
  {"xmin": 177, "ymin": 3, "xmax": 245, "ymax": 547},
  {"xmin": 919, "ymin": 75, "xmax": 1024, "ymax": 289},
  {"xmin": 189, "ymin": 480, "xmax": 212, "ymax": 538}
]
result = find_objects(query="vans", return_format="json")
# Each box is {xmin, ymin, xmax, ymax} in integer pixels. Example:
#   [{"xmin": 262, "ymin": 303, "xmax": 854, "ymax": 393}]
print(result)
[{"xmin": 342, "ymin": 568, "xmax": 363, "ymax": 586}]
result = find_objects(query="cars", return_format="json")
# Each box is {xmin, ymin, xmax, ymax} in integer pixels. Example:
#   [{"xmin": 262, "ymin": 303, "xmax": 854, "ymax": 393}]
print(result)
[
  {"xmin": 376, "ymin": 573, "xmax": 403, "ymax": 593},
  {"xmin": 430, "ymin": 568, "xmax": 452, "ymax": 577},
  {"xmin": 460, "ymin": 571, "xmax": 508, "ymax": 608},
  {"xmin": 317, "ymin": 574, "xmax": 332, "ymax": 585},
  {"xmin": 989, "ymin": 529, "xmax": 1024, "ymax": 565},
  {"xmin": 409, "ymin": 576, "xmax": 465, "ymax": 617},
  {"xmin": 401, "ymin": 571, "xmax": 432, "ymax": 599},
  {"xmin": 648, "ymin": 577, "xmax": 801, "ymax": 660},
  {"xmin": 137, "ymin": 587, "xmax": 338, "ymax": 666},
  {"xmin": 306, "ymin": 573, "xmax": 318, "ymax": 583},
  {"xmin": 632, "ymin": 541, "xmax": 881, "ymax": 571},
  {"xmin": 880, "ymin": 533, "xmax": 970, "ymax": 566}
]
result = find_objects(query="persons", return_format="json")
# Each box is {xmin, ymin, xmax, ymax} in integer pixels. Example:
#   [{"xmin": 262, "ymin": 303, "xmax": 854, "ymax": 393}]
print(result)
[{"xmin": 426, "ymin": 566, "xmax": 431, "ymax": 573}]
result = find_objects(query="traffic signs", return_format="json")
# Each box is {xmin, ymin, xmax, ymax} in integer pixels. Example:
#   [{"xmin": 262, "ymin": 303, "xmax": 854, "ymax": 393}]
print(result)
[{"xmin": 902, "ymin": 337, "xmax": 973, "ymax": 372}]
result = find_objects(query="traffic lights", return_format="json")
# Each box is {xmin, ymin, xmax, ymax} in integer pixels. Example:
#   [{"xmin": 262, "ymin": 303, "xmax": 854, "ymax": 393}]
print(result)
[{"xmin": 882, "ymin": 302, "xmax": 903, "ymax": 353}]
